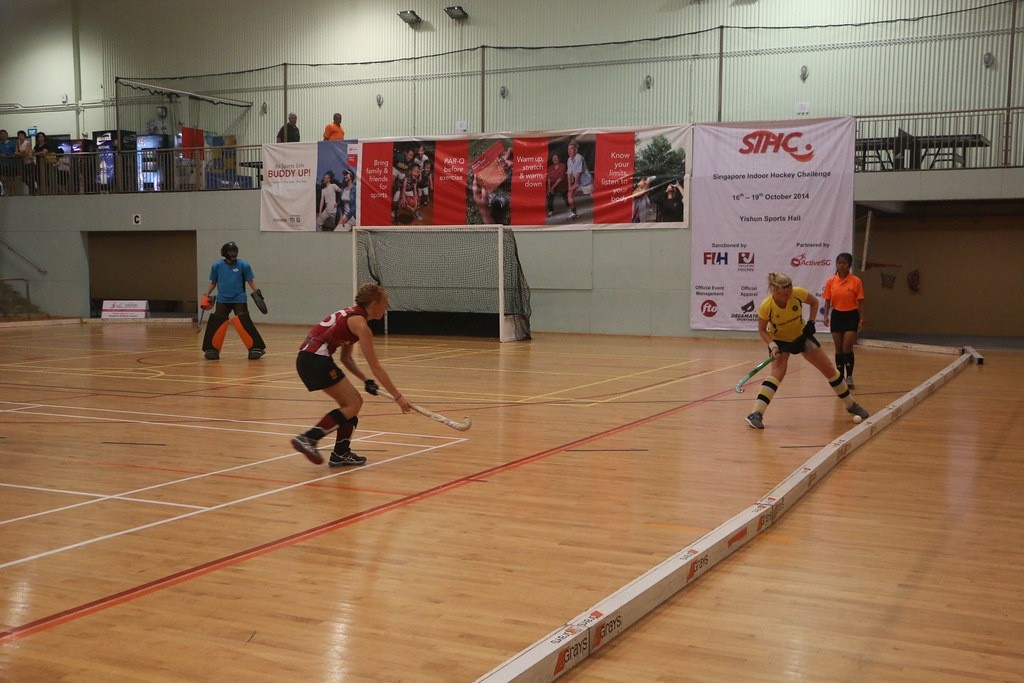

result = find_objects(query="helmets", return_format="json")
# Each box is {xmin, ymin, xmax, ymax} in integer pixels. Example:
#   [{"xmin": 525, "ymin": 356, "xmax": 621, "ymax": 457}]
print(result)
[{"xmin": 221, "ymin": 242, "xmax": 238, "ymax": 258}]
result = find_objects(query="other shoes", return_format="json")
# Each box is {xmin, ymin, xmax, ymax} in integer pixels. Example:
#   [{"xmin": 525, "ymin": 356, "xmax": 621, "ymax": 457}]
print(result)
[
  {"xmin": 548, "ymin": 211, "xmax": 554, "ymax": 217},
  {"xmin": 248, "ymin": 349, "xmax": 263, "ymax": 360},
  {"xmin": 566, "ymin": 213, "xmax": 577, "ymax": 221},
  {"xmin": 415, "ymin": 211, "xmax": 423, "ymax": 221},
  {"xmin": 204, "ymin": 348, "xmax": 219, "ymax": 361}
]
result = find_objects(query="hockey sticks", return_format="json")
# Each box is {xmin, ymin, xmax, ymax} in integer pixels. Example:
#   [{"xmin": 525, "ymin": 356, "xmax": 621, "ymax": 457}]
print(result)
[
  {"xmin": 375, "ymin": 387, "xmax": 472, "ymax": 432},
  {"xmin": 196, "ymin": 308, "xmax": 205, "ymax": 334},
  {"xmin": 735, "ymin": 331, "xmax": 809, "ymax": 393}
]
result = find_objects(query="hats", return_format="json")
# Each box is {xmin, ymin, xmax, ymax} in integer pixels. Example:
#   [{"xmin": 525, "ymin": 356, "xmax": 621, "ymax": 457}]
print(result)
[{"xmin": 342, "ymin": 168, "xmax": 354, "ymax": 180}]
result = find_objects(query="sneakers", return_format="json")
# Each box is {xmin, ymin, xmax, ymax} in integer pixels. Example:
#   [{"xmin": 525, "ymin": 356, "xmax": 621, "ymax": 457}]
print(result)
[
  {"xmin": 328, "ymin": 449, "xmax": 367, "ymax": 466},
  {"xmin": 845, "ymin": 376, "xmax": 854, "ymax": 389},
  {"xmin": 291, "ymin": 434, "xmax": 322, "ymax": 465},
  {"xmin": 745, "ymin": 411, "xmax": 765, "ymax": 429},
  {"xmin": 846, "ymin": 401, "xmax": 869, "ymax": 420}
]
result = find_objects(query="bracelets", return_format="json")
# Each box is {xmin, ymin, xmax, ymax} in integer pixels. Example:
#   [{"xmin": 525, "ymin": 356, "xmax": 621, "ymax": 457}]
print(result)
[
  {"xmin": 394, "ymin": 394, "xmax": 401, "ymax": 401},
  {"xmin": 768, "ymin": 342, "xmax": 778, "ymax": 350},
  {"xmin": 860, "ymin": 319, "xmax": 866, "ymax": 323}
]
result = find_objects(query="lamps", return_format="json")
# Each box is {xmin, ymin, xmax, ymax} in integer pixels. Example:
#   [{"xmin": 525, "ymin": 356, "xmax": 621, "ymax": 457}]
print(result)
[
  {"xmin": 984, "ymin": 52, "xmax": 994, "ymax": 68},
  {"xmin": 262, "ymin": 101, "xmax": 270, "ymax": 114},
  {"xmin": 376, "ymin": 93, "xmax": 382, "ymax": 106},
  {"xmin": 397, "ymin": 10, "xmax": 421, "ymax": 24},
  {"xmin": 443, "ymin": 6, "xmax": 468, "ymax": 20},
  {"xmin": 155, "ymin": 107, "xmax": 167, "ymax": 118},
  {"xmin": 800, "ymin": 66, "xmax": 808, "ymax": 80},
  {"xmin": 646, "ymin": 75, "xmax": 652, "ymax": 89},
  {"xmin": 500, "ymin": 86, "xmax": 508, "ymax": 99}
]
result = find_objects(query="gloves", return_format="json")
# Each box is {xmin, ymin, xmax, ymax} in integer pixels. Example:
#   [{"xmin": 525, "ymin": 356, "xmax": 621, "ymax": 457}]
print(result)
[
  {"xmin": 802, "ymin": 321, "xmax": 816, "ymax": 335},
  {"xmin": 365, "ymin": 378, "xmax": 378, "ymax": 396}
]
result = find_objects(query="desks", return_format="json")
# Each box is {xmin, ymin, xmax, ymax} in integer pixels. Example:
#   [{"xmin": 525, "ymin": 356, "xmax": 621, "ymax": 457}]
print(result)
[
  {"xmin": 853, "ymin": 128, "xmax": 991, "ymax": 170},
  {"xmin": 239, "ymin": 161, "xmax": 264, "ymax": 190}
]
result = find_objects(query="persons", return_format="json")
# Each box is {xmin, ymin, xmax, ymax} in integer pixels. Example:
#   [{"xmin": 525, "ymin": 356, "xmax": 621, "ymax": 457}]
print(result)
[
  {"xmin": 566, "ymin": 142, "xmax": 595, "ymax": 219},
  {"xmin": 15, "ymin": 131, "xmax": 39, "ymax": 195},
  {"xmin": 0, "ymin": 130, "xmax": 17, "ymax": 195},
  {"xmin": 276, "ymin": 113, "xmax": 301, "ymax": 143},
  {"xmin": 322, "ymin": 113, "xmax": 344, "ymax": 140},
  {"xmin": 319, "ymin": 169, "xmax": 356, "ymax": 231},
  {"xmin": 821, "ymin": 252, "xmax": 867, "ymax": 388},
  {"xmin": 290, "ymin": 283, "xmax": 414, "ymax": 467},
  {"xmin": 201, "ymin": 242, "xmax": 269, "ymax": 361},
  {"xmin": 546, "ymin": 153, "xmax": 573, "ymax": 217},
  {"xmin": 31, "ymin": 132, "xmax": 50, "ymax": 194},
  {"xmin": 632, "ymin": 175, "xmax": 685, "ymax": 223},
  {"xmin": 745, "ymin": 271, "xmax": 870, "ymax": 429},
  {"xmin": 392, "ymin": 144, "xmax": 434, "ymax": 221},
  {"xmin": 472, "ymin": 147, "xmax": 513, "ymax": 224}
]
